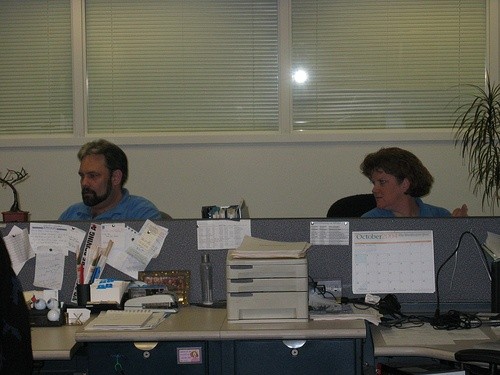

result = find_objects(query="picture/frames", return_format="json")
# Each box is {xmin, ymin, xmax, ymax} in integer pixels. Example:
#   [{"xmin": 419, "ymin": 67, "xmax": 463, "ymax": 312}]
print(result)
[{"xmin": 137, "ymin": 269, "xmax": 190, "ymax": 309}]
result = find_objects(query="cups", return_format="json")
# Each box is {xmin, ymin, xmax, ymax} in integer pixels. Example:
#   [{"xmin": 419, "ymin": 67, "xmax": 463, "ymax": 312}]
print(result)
[{"xmin": 76, "ymin": 283, "xmax": 90, "ymax": 307}]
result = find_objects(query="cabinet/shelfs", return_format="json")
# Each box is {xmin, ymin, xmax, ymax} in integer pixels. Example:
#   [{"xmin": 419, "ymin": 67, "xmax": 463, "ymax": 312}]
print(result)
[{"xmin": 69, "ymin": 302, "xmax": 367, "ymax": 375}]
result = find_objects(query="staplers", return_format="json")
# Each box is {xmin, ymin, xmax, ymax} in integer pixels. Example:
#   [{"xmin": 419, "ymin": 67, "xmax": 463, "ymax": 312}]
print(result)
[{"xmin": 124, "ymin": 294, "xmax": 180, "ymax": 313}]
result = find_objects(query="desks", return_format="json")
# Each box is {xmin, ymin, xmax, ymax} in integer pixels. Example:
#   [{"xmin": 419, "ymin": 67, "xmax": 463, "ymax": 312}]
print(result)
[
  {"xmin": 31, "ymin": 313, "xmax": 87, "ymax": 375},
  {"xmin": 370, "ymin": 313, "xmax": 500, "ymax": 375}
]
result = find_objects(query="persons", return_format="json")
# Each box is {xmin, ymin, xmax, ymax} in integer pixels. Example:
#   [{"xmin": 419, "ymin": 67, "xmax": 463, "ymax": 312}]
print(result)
[
  {"xmin": 355, "ymin": 147, "xmax": 453, "ymax": 218},
  {"xmin": 56, "ymin": 138, "xmax": 162, "ymax": 221}
]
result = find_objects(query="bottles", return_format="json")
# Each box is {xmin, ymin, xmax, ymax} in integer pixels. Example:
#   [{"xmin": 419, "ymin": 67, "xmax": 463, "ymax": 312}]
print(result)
[{"xmin": 200, "ymin": 254, "xmax": 214, "ymax": 305}]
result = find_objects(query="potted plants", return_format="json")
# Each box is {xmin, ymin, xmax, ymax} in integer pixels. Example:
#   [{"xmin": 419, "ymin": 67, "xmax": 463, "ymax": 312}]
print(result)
[{"xmin": 0, "ymin": 167, "xmax": 30, "ymax": 222}]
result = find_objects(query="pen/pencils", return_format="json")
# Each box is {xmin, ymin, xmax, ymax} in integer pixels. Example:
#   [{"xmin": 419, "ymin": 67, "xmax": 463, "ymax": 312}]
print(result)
[
  {"xmin": 80, "ymin": 263, "xmax": 85, "ymax": 286},
  {"xmin": 90, "ymin": 267, "xmax": 100, "ymax": 285}
]
